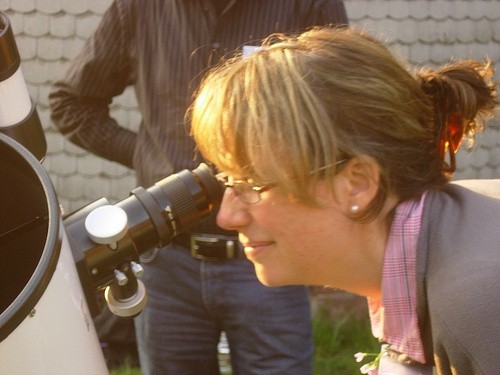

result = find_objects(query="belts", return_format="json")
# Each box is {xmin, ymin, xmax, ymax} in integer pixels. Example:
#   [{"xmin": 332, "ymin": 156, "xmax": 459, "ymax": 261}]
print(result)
[{"xmin": 172, "ymin": 232, "xmax": 239, "ymax": 261}]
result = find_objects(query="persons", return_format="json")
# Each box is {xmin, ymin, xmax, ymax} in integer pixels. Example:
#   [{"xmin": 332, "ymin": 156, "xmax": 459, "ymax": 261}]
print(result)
[
  {"xmin": 49, "ymin": 0, "xmax": 349, "ymax": 375},
  {"xmin": 182, "ymin": 24, "xmax": 500, "ymax": 375}
]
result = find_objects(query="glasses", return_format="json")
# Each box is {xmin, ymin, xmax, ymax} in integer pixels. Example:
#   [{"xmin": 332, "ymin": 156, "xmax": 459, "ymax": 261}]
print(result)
[{"xmin": 214, "ymin": 158, "xmax": 348, "ymax": 204}]
